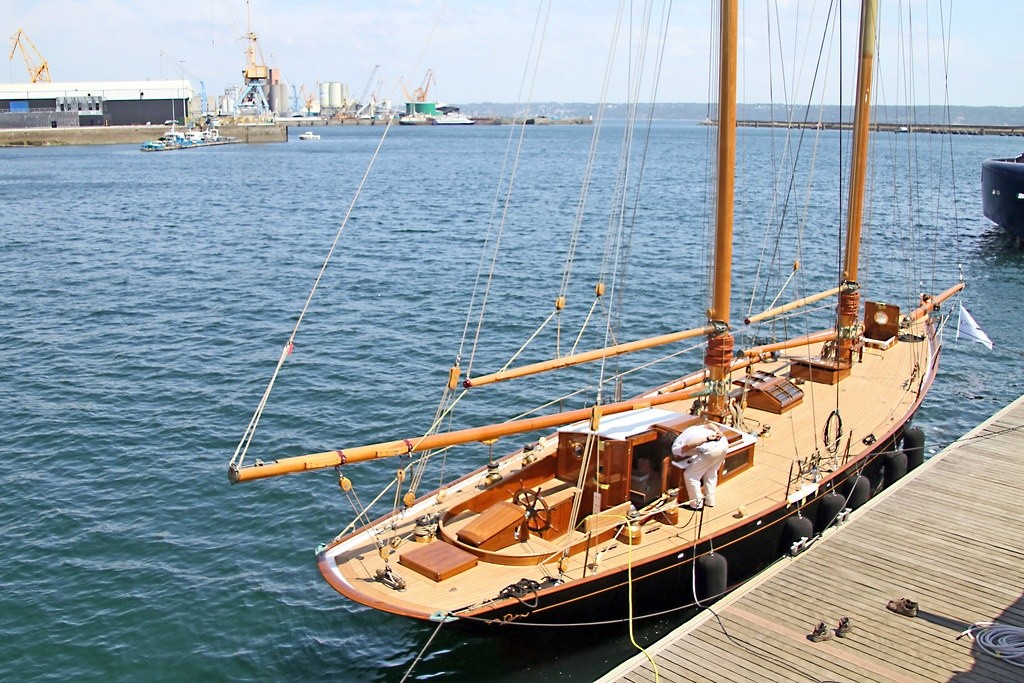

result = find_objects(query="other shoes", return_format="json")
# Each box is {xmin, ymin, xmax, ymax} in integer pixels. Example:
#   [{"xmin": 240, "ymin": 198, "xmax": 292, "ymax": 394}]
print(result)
[{"xmin": 887, "ymin": 597, "xmax": 919, "ymax": 616}]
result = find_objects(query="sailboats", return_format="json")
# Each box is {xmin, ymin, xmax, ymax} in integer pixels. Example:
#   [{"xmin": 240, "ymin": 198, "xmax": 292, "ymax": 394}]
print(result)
[
  {"xmin": 139, "ymin": 83, "xmax": 219, "ymax": 152},
  {"xmin": 221, "ymin": 2, "xmax": 969, "ymax": 626}
]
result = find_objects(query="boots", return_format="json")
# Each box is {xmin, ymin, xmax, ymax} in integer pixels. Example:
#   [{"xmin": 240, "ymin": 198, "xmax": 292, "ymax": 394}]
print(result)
[
  {"xmin": 811, "ymin": 622, "xmax": 835, "ymax": 642},
  {"xmin": 837, "ymin": 616, "xmax": 854, "ymax": 638}
]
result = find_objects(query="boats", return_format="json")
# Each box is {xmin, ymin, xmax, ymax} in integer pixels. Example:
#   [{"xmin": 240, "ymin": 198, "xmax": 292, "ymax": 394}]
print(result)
[
  {"xmin": 298, "ymin": 132, "xmax": 321, "ymax": 142},
  {"xmin": 980, "ymin": 152, "xmax": 1024, "ymax": 244},
  {"xmin": 397, "ymin": 111, "xmax": 435, "ymax": 125},
  {"xmin": 435, "ymin": 110, "xmax": 477, "ymax": 125}
]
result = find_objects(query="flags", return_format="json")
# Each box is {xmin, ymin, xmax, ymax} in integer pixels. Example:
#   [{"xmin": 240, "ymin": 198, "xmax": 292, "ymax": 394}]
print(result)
[{"xmin": 956, "ymin": 304, "xmax": 993, "ymax": 352}]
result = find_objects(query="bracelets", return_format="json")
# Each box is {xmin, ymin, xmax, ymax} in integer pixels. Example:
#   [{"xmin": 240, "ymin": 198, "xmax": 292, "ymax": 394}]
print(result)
[{"xmin": 707, "ymin": 437, "xmax": 710, "ymax": 442}]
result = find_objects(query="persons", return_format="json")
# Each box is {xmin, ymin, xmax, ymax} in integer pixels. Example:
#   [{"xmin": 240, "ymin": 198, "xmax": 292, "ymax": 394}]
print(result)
[{"xmin": 666, "ymin": 424, "xmax": 729, "ymax": 512}]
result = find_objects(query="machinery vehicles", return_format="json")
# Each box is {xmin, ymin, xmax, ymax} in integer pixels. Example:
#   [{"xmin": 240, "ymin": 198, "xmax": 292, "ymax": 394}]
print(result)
[{"xmin": 9, "ymin": 28, "xmax": 52, "ymax": 83}]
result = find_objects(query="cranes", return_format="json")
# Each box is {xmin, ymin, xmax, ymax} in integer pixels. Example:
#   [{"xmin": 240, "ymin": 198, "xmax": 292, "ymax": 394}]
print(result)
[
  {"xmin": 400, "ymin": 68, "xmax": 436, "ymax": 102},
  {"xmin": 289, "ymin": 83, "xmax": 318, "ymax": 116},
  {"xmin": 225, "ymin": 2, "xmax": 278, "ymax": 118}
]
